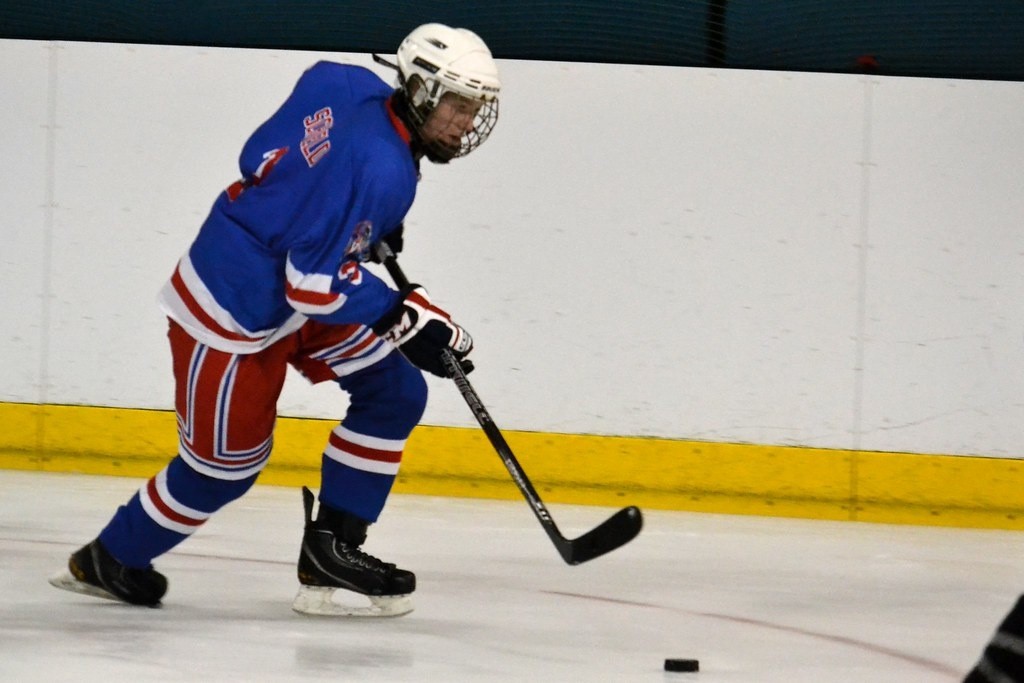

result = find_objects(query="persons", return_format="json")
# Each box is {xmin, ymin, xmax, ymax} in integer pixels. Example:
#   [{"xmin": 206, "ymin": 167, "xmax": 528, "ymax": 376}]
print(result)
[{"xmin": 70, "ymin": 21, "xmax": 505, "ymax": 608}]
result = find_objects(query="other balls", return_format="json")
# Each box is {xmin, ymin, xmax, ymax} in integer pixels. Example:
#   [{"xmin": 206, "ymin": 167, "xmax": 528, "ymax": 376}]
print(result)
[{"xmin": 664, "ymin": 654, "xmax": 702, "ymax": 677}]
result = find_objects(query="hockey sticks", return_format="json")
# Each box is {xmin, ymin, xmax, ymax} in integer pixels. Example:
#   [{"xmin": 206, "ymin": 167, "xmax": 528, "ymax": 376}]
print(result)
[{"xmin": 371, "ymin": 239, "xmax": 644, "ymax": 567}]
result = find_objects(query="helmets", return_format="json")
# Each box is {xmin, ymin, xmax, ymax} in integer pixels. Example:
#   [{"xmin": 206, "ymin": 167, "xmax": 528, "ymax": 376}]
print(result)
[{"xmin": 374, "ymin": 23, "xmax": 501, "ymax": 162}]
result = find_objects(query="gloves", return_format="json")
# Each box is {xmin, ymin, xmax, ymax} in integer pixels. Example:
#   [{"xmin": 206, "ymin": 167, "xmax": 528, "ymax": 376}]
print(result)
[{"xmin": 373, "ymin": 283, "xmax": 474, "ymax": 379}]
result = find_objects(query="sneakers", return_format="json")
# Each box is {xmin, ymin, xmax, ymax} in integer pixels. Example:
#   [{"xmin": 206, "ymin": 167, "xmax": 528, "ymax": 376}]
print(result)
[
  {"xmin": 292, "ymin": 486, "xmax": 416, "ymax": 618},
  {"xmin": 47, "ymin": 537, "xmax": 168, "ymax": 609}
]
